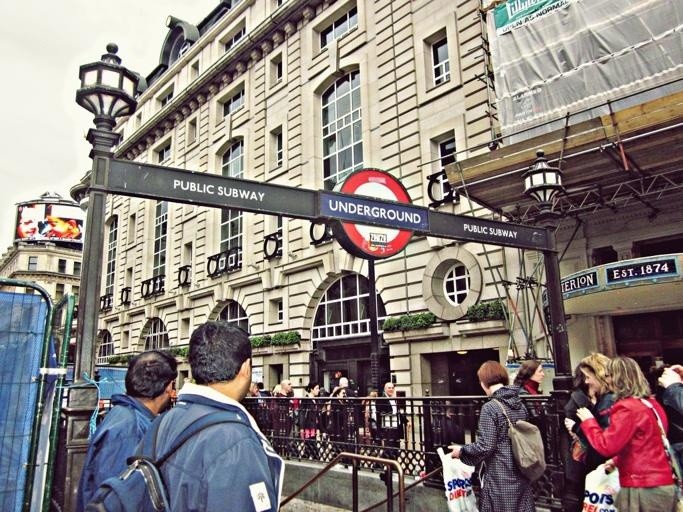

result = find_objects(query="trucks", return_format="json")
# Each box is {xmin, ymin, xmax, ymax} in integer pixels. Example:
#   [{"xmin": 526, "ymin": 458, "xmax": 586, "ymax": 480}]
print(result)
[{"xmin": 64, "ymin": 365, "xmax": 129, "ymax": 414}]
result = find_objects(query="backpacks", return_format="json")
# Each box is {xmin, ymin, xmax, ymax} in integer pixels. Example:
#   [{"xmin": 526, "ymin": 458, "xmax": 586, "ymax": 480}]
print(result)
[{"xmin": 83, "ymin": 409, "xmax": 250, "ymax": 512}]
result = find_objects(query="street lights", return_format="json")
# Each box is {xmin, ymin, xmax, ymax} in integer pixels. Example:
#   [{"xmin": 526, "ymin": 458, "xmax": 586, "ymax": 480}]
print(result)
[
  {"xmin": 71, "ymin": 42, "xmax": 145, "ymax": 384},
  {"xmin": 522, "ymin": 149, "xmax": 572, "ymax": 375}
]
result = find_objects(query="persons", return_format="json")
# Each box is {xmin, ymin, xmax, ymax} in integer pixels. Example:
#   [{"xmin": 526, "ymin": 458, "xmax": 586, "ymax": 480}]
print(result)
[
  {"xmin": 245, "ymin": 372, "xmax": 416, "ymax": 473},
  {"xmin": 444, "ymin": 359, "xmax": 539, "ymax": 512},
  {"xmin": 132, "ymin": 320, "xmax": 284, "ymax": 512},
  {"xmin": 73, "ymin": 348, "xmax": 178, "ymax": 512},
  {"xmin": 563, "ymin": 352, "xmax": 615, "ymax": 512},
  {"xmin": 573, "ymin": 356, "xmax": 678, "ymax": 512},
  {"xmin": 17, "ymin": 205, "xmax": 38, "ymax": 239},
  {"xmin": 46, "ymin": 203, "xmax": 82, "ymax": 240},
  {"xmin": 652, "ymin": 363, "xmax": 682, "ymax": 418},
  {"xmin": 513, "ymin": 358, "xmax": 563, "ymax": 512},
  {"xmin": 559, "ymin": 363, "xmax": 600, "ymax": 512}
]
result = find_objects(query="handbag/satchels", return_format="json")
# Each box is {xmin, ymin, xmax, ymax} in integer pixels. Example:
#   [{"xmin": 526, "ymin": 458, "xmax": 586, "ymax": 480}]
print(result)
[
  {"xmin": 571, "ymin": 439, "xmax": 588, "ymax": 466},
  {"xmin": 437, "ymin": 446, "xmax": 480, "ymax": 512},
  {"xmin": 492, "ymin": 399, "xmax": 546, "ymax": 482},
  {"xmin": 582, "ymin": 461, "xmax": 621, "ymax": 512}
]
